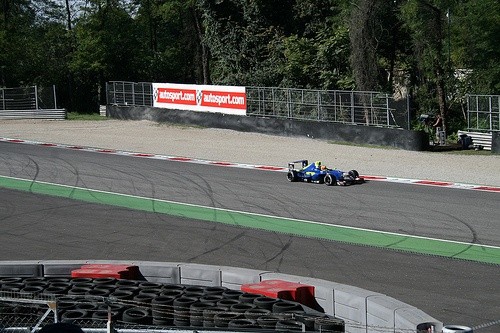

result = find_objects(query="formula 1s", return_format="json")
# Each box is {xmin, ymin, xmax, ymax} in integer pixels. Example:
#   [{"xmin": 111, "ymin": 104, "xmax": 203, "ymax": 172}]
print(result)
[{"xmin": 286, "ymin": 158, "xmax": 360, "ymax": 186}]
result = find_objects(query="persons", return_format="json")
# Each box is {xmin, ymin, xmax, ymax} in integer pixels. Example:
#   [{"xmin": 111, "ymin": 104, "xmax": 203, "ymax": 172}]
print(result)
[
  {"xmin": 315, "ymin": 161, "xmax": 322, "ymax": 170},
  {"xmin": 363, "ymin": 109, "xmax": 371, "ymax": 123},
  {"xmin": 433, "ymin": 113, "xmax": 443, "ymax": 141}
]
